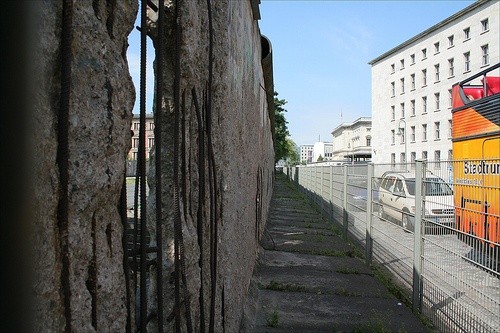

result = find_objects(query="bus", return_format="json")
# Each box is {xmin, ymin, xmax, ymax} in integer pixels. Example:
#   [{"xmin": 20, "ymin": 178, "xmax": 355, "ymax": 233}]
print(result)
[{"xmin": 450, "ymin": 62, "xmax": 500, "ymax": 258}]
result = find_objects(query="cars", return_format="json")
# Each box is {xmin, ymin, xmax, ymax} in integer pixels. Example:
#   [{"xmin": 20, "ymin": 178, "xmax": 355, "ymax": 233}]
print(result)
[{"xmin": 341, "ymin": 178, "xmax": 379, "ymax": 211}]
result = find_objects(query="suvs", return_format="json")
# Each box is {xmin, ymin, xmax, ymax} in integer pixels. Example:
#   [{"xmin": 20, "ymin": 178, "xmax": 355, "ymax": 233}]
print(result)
[{"xmin": 378, "ymin": 170, "xmax": 453, "ymax": 232}]
